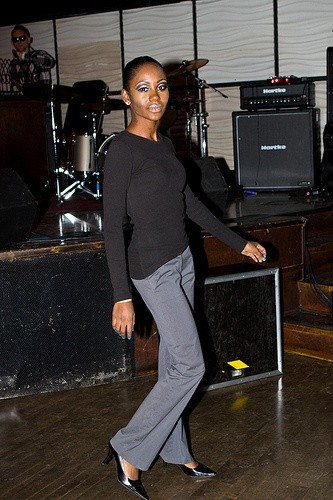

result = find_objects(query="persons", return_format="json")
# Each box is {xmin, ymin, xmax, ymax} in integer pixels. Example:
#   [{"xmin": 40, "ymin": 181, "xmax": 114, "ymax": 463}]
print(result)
[
  {"xmin": 101, "ymin": 56, "xmax": 266, "ymax": 500},
  {"xmin": 9, "ymin": 24, "xmax": 50, "ymax": 83}
]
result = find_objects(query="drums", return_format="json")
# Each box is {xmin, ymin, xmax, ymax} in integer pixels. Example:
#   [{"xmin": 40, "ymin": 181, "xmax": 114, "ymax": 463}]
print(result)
[
  {"xmin": 60, "ymin": 132, "xmax": 95, "ymax": 173},
  {"xmin": 95, "ymin": 134, "xmax": 117, "ymax": 186}
]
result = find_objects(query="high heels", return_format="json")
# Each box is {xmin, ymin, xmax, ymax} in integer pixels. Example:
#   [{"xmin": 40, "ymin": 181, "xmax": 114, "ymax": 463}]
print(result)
[
  {"xmin": 102, "ymin": 440, "xmax": 150, "ymax": 500},
  {"xmin": 163, "ymin": 453, "xmax": 217, "ymax": 478}
]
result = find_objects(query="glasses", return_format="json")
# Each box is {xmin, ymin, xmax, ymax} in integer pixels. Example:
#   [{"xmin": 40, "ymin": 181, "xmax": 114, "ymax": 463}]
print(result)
[{"xmin": 12, "ymin": 35, "xmax": 28, "ymax": 43}]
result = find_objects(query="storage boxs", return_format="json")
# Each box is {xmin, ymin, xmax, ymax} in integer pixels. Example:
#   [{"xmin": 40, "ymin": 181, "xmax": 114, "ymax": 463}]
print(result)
[{"xmin": 195, "ymin": 262, "xmax": 284, "ymax": 392}]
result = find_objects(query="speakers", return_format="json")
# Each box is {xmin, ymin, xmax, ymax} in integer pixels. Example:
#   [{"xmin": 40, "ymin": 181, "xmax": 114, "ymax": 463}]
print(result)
[
  {"xmin": 0, "ymin": 168, "xmax": 38, "ymax": 245},
  {"xmin": 231, "ymin": 107, "xmax": 321, "ymax": 191},
  {"xmin": 194, "ymin": 263, "xmax": 284, "ymax": 394}
]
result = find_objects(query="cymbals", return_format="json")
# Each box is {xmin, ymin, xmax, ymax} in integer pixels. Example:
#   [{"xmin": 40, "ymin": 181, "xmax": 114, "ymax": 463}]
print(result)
[{"xmin": 167, "ymin": 59, "xmax": 209, "ymax": 77}]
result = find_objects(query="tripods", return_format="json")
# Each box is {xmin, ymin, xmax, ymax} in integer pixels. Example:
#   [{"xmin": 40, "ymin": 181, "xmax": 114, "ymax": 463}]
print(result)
[{"xmin": 38, "ymin": 88, "xmax": 97, "ymax": 206}]
